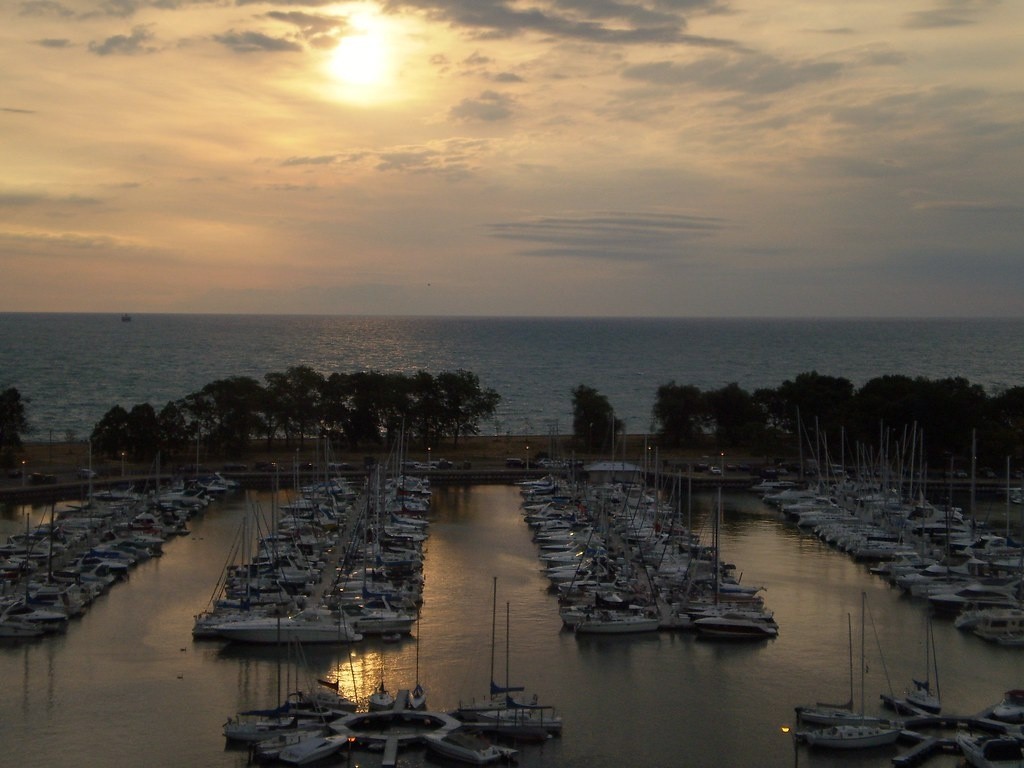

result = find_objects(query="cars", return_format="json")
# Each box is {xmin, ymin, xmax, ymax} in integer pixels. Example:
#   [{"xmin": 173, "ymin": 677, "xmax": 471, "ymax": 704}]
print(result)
[
  {"xmin": 75, "ymin": 469, "xmax": 99, "ymax": 480},
  {"xmin": 27, "ymin": 474, "xmax": 55, "ymax": 482},
  {"xmin": 223, "ymin": 461, "xmax": 247, "ymax": 471},
  {"xmin": 9, "ymin": 469, "xmax": 25, "ymax": 479},
  {"xmin": 257, "ymin": 462, "xmax": 287, "ymax": 472},
  {"xmin": 297, "ymin": 460, "xmax": 471, "ymax": 470}
]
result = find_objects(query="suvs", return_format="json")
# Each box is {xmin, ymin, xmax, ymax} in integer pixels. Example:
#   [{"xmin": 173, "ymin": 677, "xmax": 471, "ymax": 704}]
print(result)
[{"xmin": 506, "ymin": 458, "xmax": 526, "ymax": 468}]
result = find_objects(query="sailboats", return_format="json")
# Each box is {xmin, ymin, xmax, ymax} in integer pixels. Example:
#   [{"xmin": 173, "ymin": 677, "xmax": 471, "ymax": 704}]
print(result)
[
  {"xmin": 195, "ymin": 412, "xmax": 434, "ymax": 644},
  {"xmin": 221, "ymin": 574, "xmax": 565, "ymax": 768},
  {"xmin": 751, "ymin": 402, "xmax": 1023, "ymax": 646},
  {"xmin": 791, "ymin": 587, "xmax": 1024, "ymax": 768},
  {"xmin": 516, "ymin": 409, "xmax": 779, "ymax": 638},
  {"xmin": 0, "ymin": 424, "xmax": 238, "ymax": 640}
]
